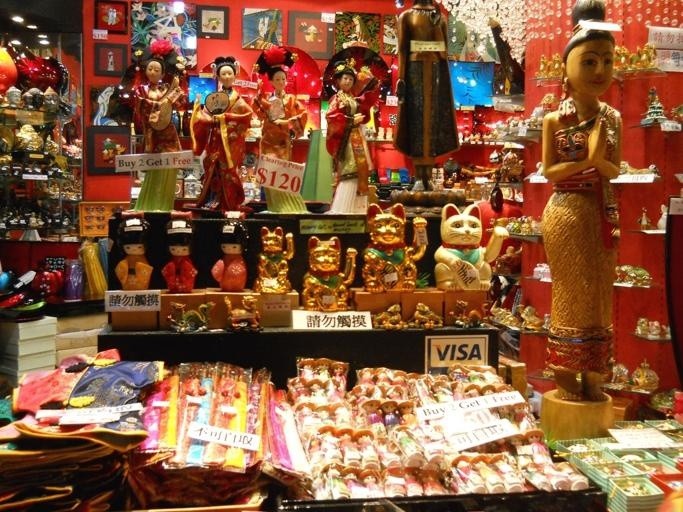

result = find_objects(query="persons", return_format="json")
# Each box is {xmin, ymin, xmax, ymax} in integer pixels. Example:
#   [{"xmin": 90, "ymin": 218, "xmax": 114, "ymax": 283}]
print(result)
[
  {"xmin": 295, "ymin": 358, "xmax": 469, "ymax": 501},
  {"xmin": 210, "ymin": 221, "xmax": 249, "ymax": 291},
  {"xmin": 191, "ymin": 56, "xmax": 254, "ymax": 215},
  {"xmin": 452, "ymin": 364, "xmax": 585, "ymax": 494},
  {"xmin": 254, "ymin": 47, "xmax": 310, "ymax": 208},
  {"xmin": 542, "ymin": 1, "xmax": 623, "ymax": 403},
  {"xmin": 128, "ymin": 43, "xmax": 190, "ymax": 210},
  {"xmin": 80, "ymin": 237, "xmax": 106, "ymax": 303},
  {"xmin": 397, "ymin": 0, "xmax": 455, "ymax": 189},
  {"xmin": 322, "ymin": 60, "xmax": 382, "ymax": 214},
  {"xmin": 111, "ymin": 219, "xmax": 153, "ymax": 292},
  {"xmin": 161, "ymin": 220, "xmax": 199, "ymax": 294}
]
